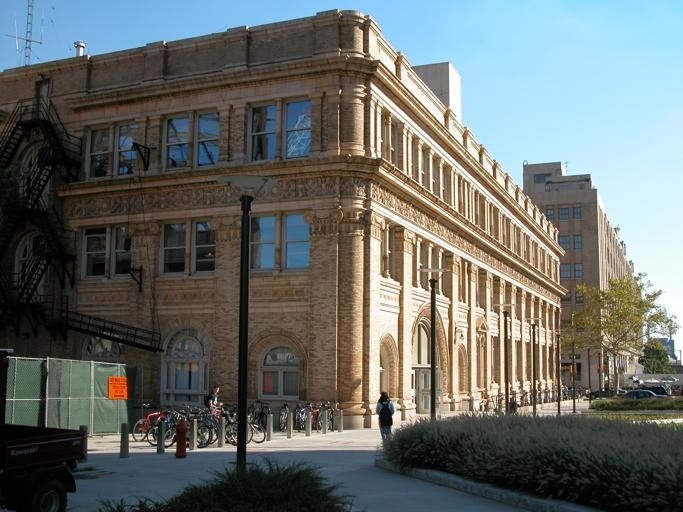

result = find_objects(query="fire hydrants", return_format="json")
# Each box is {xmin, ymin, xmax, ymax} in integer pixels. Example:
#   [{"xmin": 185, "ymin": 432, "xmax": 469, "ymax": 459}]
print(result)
[{"xmin": 172, "ymin": 419, "xmax": 187, "ymax": 459}]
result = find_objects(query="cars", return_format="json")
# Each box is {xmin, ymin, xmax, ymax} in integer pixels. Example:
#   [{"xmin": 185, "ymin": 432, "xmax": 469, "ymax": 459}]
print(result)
[{"xmin": 584, "ymin": 376, "xmax": 679, "ymax": 401}]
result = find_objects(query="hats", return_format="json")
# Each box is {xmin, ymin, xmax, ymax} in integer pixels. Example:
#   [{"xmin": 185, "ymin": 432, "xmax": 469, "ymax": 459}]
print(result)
[{"xmin": 380, "ymin": 391, "xmax": 388, "ymax": 396}]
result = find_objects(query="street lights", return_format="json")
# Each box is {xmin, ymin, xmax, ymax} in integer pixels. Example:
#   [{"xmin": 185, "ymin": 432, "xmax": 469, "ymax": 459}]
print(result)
[
  {"xmin": 421, "ymin": 267, "xmax": 450, "ymax": 418},
  {"xmin": 217, "ymin": 174, "xmax": 271, "ymax": 479},
  {"xmin": 552, "ymin": 327, "xmax": 561, "ymax": 416},
  {"xmin": 493, "ymin": 302, "xmax": 515, "ymax": 413},
  {"xmin": 525, "ymin": 317, "xmax": 543, "ymax": 418}
]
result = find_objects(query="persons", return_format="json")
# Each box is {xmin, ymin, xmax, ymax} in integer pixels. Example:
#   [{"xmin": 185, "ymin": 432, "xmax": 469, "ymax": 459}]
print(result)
[
  {"xmin": 202, "ymin": 384, "xmax": 223, "ymax": 416},
  {"xmin": 376, "ymin": 390, "xmax": 395, "ymax": 447},
  {"xmin": 666, "ymin": 385, "xmax": 671, "ymax": 395}
]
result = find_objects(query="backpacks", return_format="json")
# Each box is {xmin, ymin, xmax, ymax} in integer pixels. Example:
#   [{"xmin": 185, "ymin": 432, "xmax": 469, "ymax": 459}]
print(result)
[{"xmin": 378, "ymin": 400, "xmax": 393, "ymax": 427}]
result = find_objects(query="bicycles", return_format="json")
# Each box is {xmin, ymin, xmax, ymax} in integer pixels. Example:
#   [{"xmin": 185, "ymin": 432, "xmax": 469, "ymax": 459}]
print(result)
[{"xmin": 132, "ymin": 400, "xmax": 341, "ymax": 449}]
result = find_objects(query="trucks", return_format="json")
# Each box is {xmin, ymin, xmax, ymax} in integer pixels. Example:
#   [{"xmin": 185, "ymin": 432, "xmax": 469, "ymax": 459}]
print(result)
[{"xmin": 1, "ymin": 422, "xmax": 86, "ymax": 512}]
